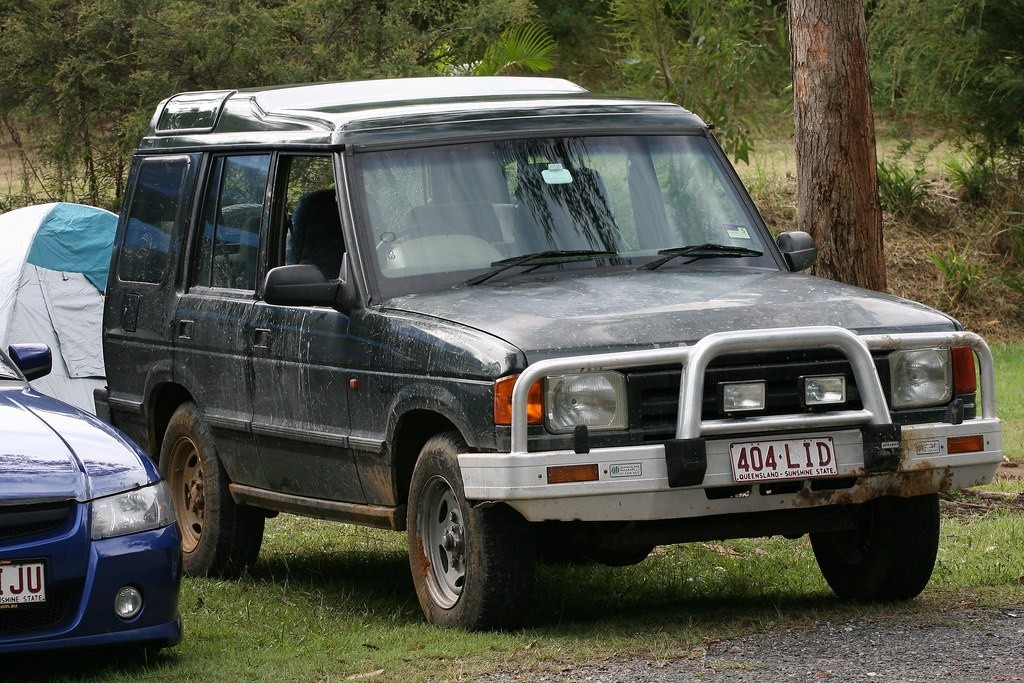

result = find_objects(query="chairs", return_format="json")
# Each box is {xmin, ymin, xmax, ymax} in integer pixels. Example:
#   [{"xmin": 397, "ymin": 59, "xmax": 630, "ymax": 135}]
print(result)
[
  {"xmin": 401, "ymin": 202, "xmax": 461, "ymax": 244},
  {"xmin": 287, "ymin": 189, "xmax": 387, "ymax": 282}
]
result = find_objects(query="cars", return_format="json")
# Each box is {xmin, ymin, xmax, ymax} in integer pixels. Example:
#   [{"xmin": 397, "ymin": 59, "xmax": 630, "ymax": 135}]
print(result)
[{"xmin": 0, "ymin": 342, "xmax": 184, "ymax": 653}]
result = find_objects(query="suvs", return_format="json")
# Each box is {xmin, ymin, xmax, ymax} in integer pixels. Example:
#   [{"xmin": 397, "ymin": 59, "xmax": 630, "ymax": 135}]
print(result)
[{"xmin": 91, "ymin": 74, "xmax": 1003, "ymax": 632}]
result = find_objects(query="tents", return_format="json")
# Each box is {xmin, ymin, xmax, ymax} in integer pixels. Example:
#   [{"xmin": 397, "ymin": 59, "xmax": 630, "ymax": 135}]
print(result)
[{"xmin": 0, "ymin": 202, "xmax": 171, "ymax": 415}]
